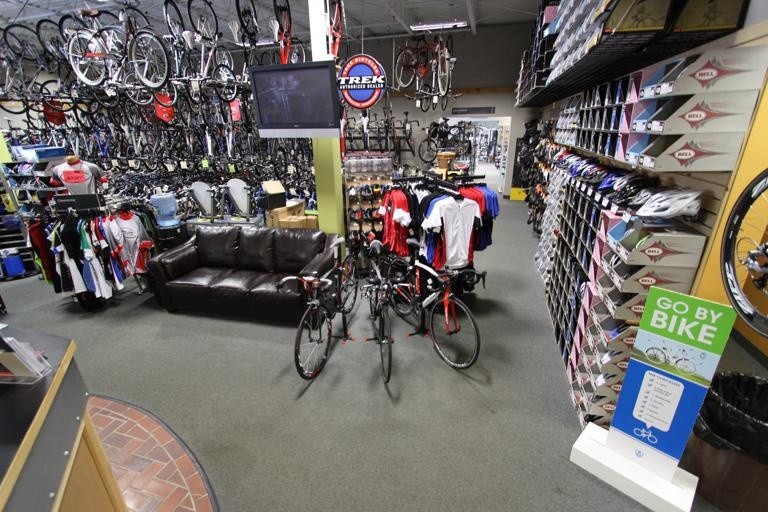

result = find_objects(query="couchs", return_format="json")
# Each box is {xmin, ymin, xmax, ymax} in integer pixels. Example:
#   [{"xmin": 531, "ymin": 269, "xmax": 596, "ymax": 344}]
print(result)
[{"xmin": 146, "ymin": 224, "xmax": 341, "ymax": 324}]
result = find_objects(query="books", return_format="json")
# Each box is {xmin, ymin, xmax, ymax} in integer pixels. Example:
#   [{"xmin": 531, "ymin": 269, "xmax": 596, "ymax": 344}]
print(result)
[{"xmin": 0, "ymin": 335, "xmax": 46, "ymax": 377}]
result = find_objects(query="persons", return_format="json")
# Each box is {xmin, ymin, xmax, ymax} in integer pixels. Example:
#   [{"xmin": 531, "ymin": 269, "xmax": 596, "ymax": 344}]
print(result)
[{"xmin": 49, "ymin": 155, "xmax": 108, "ymax": 195}]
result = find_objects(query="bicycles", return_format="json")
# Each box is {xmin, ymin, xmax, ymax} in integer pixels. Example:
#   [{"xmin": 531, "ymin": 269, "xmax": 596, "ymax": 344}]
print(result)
[
  {"xmin": 721, "ymin": 164, "xmax": 767, "ymax": 336},
  {"xmin": 386, "ymin": 233, "xmax": 487, "ymax": 370},
  {"xmin": 353, "ymin": 230, "xmax": 398, "ymax": 384},
  {"xmin": 644, "ymin": 345, "xmax": 697, "ymax": 377},
  {"xmin": 0, "ymin": 0, "xmax": 472, "ymax": 214},
  {"xmin": 273, "ymin": 239, "xmax": 359, "ymax": 380}
]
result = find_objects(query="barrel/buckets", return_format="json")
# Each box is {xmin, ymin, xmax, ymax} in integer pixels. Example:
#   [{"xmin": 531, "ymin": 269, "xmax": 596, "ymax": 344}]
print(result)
[{"xmin": 150, "ymin": 193, "xmax": 181, "ymax": 227}]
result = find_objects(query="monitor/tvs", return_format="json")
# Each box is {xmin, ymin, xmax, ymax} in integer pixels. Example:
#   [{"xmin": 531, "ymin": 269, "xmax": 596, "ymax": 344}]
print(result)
[{"xmin": 248, "ymin": 61, "xmax": 341, "ymax": 139}]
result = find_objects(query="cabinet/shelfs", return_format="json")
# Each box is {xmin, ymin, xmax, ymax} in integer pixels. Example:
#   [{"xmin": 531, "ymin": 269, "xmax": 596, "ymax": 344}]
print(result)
[{"xmin": 512, "ymin": 0, "xmax": 768, "ymax": 430}]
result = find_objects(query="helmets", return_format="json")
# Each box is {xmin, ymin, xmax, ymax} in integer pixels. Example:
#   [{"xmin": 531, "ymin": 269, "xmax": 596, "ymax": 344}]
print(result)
[{"xmin": 536, "ymin": 146, "xmax": 702, "ymax": 223}]
